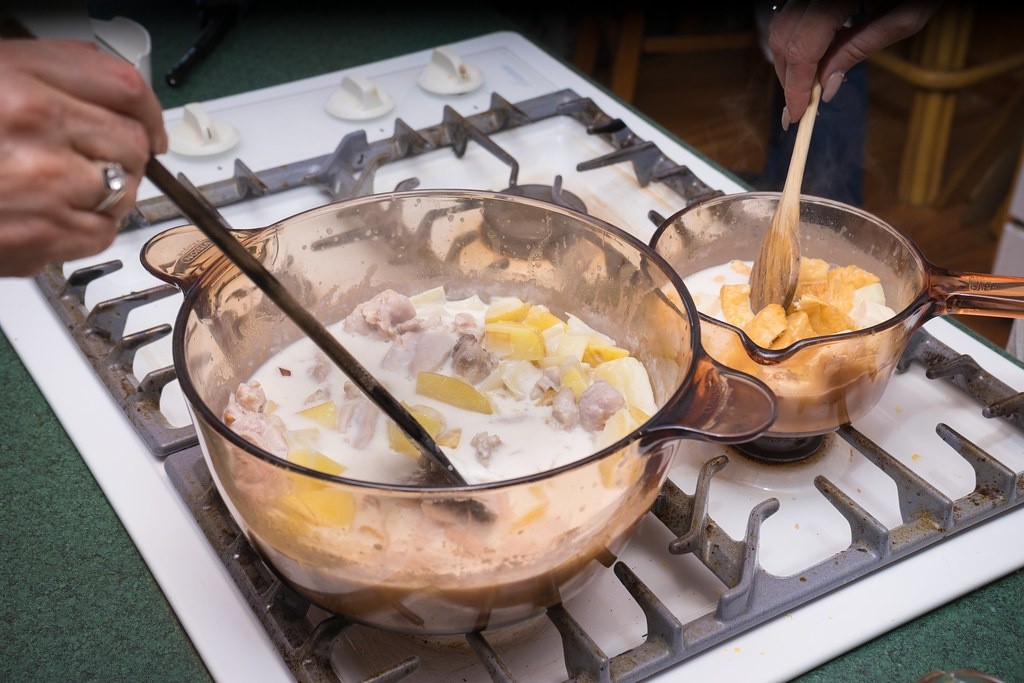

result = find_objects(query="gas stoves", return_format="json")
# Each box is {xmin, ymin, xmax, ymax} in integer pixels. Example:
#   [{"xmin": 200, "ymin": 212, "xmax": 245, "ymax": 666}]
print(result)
[{"xmin": 0, "ymin": 29, "xmax": 1024, "ymax": 683}]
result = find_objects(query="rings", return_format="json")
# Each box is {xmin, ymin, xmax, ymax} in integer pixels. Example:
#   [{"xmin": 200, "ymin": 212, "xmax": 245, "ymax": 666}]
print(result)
[
  {"xmin": 95, "ymin": 164, "xmax": 126, "ymax": 211},
  {"xmin": 768, "ymin": 2, "xmax": 782, "ymax": 14}
]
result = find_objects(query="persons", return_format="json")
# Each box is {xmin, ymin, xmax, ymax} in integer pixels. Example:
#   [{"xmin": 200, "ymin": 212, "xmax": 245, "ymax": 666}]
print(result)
[
  {"xmin": 0, "ymin": 37, "xmax": 170, "ymax": 278},
  {"xmin": 755, "ymin": 0, "xmax": 941, "ymax": 210}
]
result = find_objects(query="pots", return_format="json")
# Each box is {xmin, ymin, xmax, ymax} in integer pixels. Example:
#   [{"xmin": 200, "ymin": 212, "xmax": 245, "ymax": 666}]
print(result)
[
  {"xmin": 141, "ymin": 187, "xmax": 782, "ymax": 631},
  {"xmin": 643, "ymin": 188, "xmax": 1024, "ymax": 448}
]
